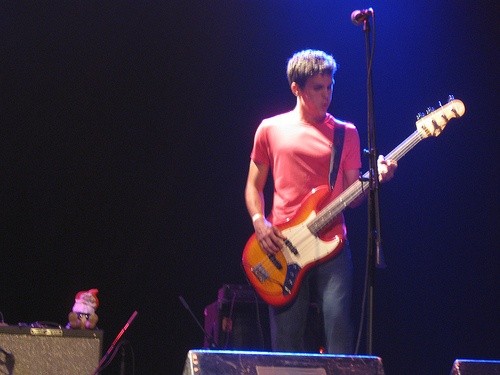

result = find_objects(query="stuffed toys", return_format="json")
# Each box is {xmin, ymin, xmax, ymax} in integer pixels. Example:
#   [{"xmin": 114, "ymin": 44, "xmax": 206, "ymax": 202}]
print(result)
[{"xmin": 67, "ymin": 288, "xmax": 100, "ymax": 330}]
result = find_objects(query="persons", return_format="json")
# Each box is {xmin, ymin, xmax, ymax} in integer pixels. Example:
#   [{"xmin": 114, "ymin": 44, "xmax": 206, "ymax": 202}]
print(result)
[{"xmin": 246, "ymin": 49, "xmax": 398, "ymax": 356}]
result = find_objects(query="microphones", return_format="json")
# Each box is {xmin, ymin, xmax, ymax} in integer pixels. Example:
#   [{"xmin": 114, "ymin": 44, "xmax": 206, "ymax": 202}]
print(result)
[{"xmin": 351, "ymin": 7, "xmax": 373, "ymax": 24}]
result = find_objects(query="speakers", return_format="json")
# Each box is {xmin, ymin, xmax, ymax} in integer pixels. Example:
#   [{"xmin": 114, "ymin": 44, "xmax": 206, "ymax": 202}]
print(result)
[
  {"xmin": 181, "ymin": 349, "xmax": 385, "ymax": 375},
  {"xmin": 0, "ymin": 326, "xmax": 104, "ymax": 375},
  {"xmin": 450, "ymin": 359, "xmax": 500, "ymax": 375}
]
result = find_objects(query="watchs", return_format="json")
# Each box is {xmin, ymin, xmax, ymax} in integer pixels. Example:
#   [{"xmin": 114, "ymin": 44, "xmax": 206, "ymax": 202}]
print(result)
[{"xmin": 252, "ymin": 213, "xmax": 266, "ymax": 222}]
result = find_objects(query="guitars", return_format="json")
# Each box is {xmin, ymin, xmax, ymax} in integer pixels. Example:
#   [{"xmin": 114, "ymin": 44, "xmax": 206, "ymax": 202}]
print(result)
[{"xmin": 242, "ymin": 94, "xmax": 465, "ymax": 306}]
